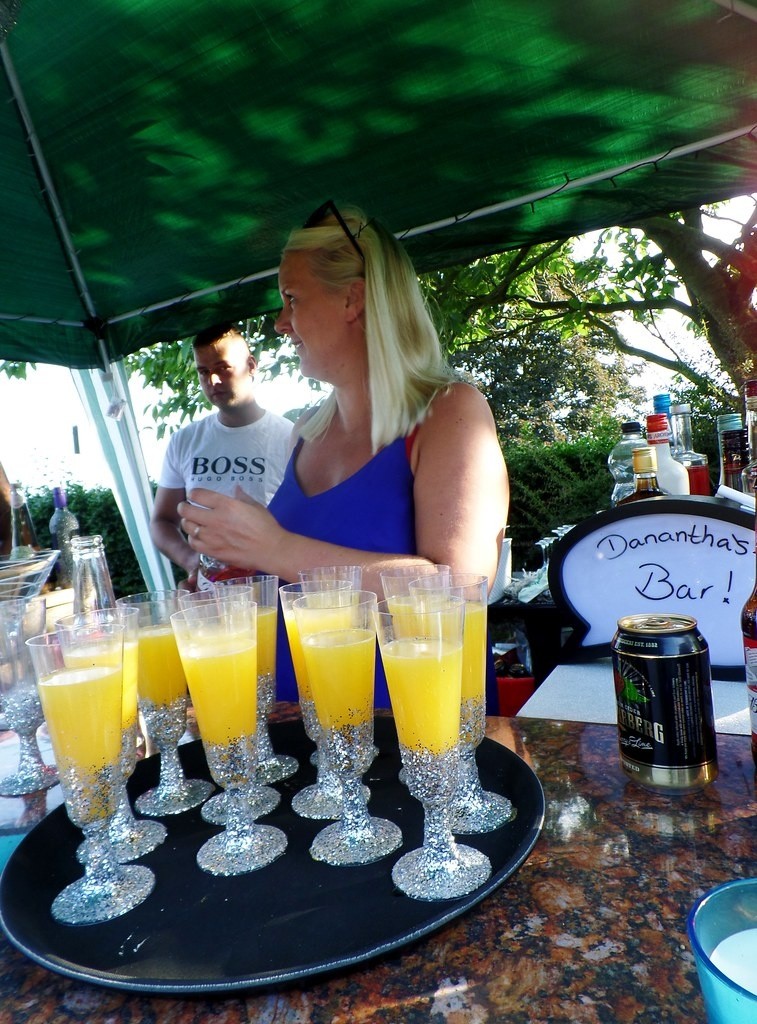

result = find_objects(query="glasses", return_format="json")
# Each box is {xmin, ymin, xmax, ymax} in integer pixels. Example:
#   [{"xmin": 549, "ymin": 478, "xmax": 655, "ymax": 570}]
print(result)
[{"xmin": 303, "ymin": 199, "xmax": 366, "ymax": 267}]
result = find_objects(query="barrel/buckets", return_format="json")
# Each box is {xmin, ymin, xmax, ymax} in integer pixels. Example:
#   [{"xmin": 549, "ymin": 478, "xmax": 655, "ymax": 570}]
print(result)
[{"xmin": 496, "ymin": 675, "xmax": 534, "ymax": 717}]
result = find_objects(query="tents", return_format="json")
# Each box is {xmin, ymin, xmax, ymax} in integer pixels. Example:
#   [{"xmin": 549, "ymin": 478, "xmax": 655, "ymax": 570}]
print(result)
[{"xmin": 0, "ymin": 0, "xmax": 755, "ymax": 730}]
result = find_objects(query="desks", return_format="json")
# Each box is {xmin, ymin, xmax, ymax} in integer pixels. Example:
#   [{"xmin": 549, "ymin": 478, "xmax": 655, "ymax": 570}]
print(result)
[
  {"xmin": 486, "ymin": 572, "xmax": 572, "ymax": 693},
  {"xmin": 0, "ymin": 702, "xmax": 757, "ymax": 1024},
  {"xmin": 514, "ymin": 657, "xmax": 751, "ymax": 736}
]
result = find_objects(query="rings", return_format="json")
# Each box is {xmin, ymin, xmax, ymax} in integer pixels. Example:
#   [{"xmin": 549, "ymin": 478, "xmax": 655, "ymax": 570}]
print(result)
[{"xmin": 190, "ymin": 526, "xmax": 201, "ymax": 537}]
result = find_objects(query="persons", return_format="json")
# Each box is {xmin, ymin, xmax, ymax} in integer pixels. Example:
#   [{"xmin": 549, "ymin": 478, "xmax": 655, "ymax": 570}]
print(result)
[
  {"xmin": 148, "ymin": 320, "xmax": 296, "ymax": 592},
  {"xmin": 176, "ymin": 199, "xmax": 509, "ymax": 612}
]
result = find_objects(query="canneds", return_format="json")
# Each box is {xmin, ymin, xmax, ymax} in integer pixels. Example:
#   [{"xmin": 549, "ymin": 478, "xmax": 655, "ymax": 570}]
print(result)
[{"xmin": 611, "ymin": 614, "xmax": 721, "ymax": 796}]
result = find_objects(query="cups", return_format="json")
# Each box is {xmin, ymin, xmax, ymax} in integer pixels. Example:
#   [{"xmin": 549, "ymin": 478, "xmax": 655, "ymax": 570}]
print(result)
[{"xmin": 687, "ymin": 879, "xmax": 757, "ymax": 1024}]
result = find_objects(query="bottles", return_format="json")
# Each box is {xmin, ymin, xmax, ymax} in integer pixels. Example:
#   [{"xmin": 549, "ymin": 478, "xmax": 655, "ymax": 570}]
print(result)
[
  {"xmin": 69, "ymin": 536, "xmax": 115, "ymax": 627},
  {"xmin": 617, "ymin": 447, "xmax": 667, "ymax": 504},
  {"xmin": 714, "ymin": 413, "xmax": 750, "ymax": 495},
  {"xmin": 741, "ymin": 466, "xmax": 757, "ymax": 763},
  {"xmin": 194, "ymin": 555, "xmax": 255, "ymax": 592},
  {"xmin": 646, "ymin": 393, "xmax": 710, "ymax": 496},
  {"xmin": 607, "ymin": 422, "xmax": 648, "ymax": 507},
  {"xmin": 741, "ymin": 380, "xmax": 757, "ymax": 497},
  {"xmin": 9, "ymin": 481, "xmax": 43, "ymax": 599},
  {"xmin": 50, "ymin": 486, "xmax": 82, "ymax": 589}
]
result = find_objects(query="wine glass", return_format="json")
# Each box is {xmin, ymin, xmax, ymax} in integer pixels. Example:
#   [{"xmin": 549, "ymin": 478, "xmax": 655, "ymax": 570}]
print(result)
[
  {"xmin": 115, "ymin": 590, "xmax": 213, "ymax": 816},
  {"xmin": 371, "ymin": 593, "xmax": 492, "ymax": 901},
  {"xmin": 407, "ymin": 572, "xmax": 511, "ymax": 836},
  {"xmin": 291, "ymin": 589, "xmax": 402, "ymax": 866},
  {"xmin": 26, "ymin": 623, "xmax": 155, "ymax": 923},
  {"xmin": 379, "ymin": 564, "xmax": 450, "ymax": 784},
  {"xmin": 170, "ymin": 602, "xmax": 288, "ymax": 876},
  {"xmin": 55, "ymin": 606, "xmax": 166, "ymax": 862},
  {"xmin": 300, "ymin": 566, "xmax": 379, "ymax": 765},
  {"xmin": 0, "ymin": 550, "xmax": 62, "ymax": 731},
  {"xmin": 215, "ymin": 575, "xmax": 299, "ymax": 784},
  {"xmin": 177, "ymin": 584, "xmax": 279, "ymax": 824},
  {"xmin": 279, "ymin": 580, "xmax": 379, "ymax": 819},
  {"xmin": 0, "ymin": 599, "xmax": 60, "ymax": 796}
]
